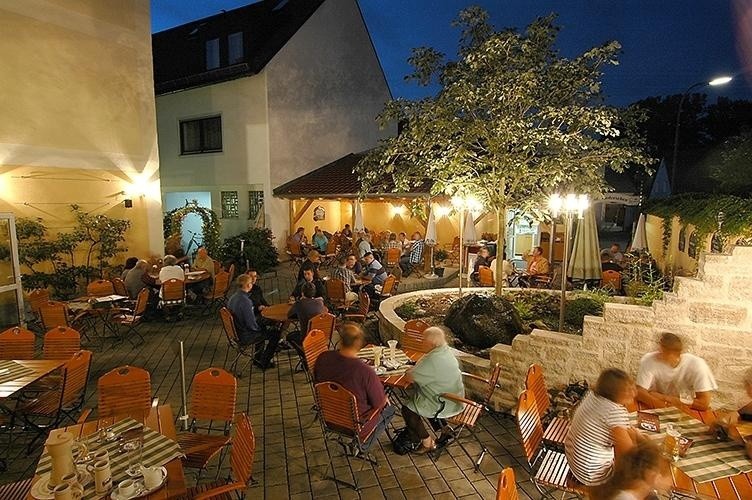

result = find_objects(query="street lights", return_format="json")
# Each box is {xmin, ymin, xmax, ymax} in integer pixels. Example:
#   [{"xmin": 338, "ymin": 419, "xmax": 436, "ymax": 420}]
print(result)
[
  {"xmin": 439, "ymin": 196, "xmax": 483, "ymax": 299},
  {"xmin": 549, "ymin": 193, "xmax": 589, "ymax": 333},
  {"xmin": 671, "ymin": 75, "xmax": 733, "ymax": 190}
]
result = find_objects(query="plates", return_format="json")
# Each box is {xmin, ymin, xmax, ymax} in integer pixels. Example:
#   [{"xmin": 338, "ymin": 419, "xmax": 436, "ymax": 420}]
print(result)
[
  {"xmin": 370, "ymin": 366, "xmax": 387, "ymax": 376},
  {"xmin": 111, "ymin": 480, "xmax": 142, "ymax": 500},
  {"xmin": 30, "ymin": 464, "xmax": 91, "ymax": 500}
]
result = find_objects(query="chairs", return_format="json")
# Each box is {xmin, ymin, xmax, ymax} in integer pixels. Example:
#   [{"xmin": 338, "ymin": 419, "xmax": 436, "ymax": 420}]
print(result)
[
  {"xmin": 200, "ymin": 273, "xmax": 228, "ymax": 320},
  {"xmin": 112, "ymin": 278, "xmax": 133, "ymax": 316},
  {"xmin": 9, "ymin": 327, "xmax": 80, "ymax": 431},
  {"xmin": 87, "ymin": 280, "xmax": 120, "ymax": 339},
  {"xmin": 601, "ymin": 270, "xmax": 622, "ymax": 295},
  {"xmin": 326, "ymin": 278, "xmax": 356, "ymax": 324},
  {"xmin": 220, "ymin": 308, "xmax": 264, "ymax": 377},
  {"xmin": 311, "ymin": 381, "xmax": 380, "ymax": 491},
  {"xmin": 526, "ymin": 363, "xmax": 572, "ymax": 455},
  {"xmin": 28, "ymin": 288, "xmax": 47, "ymax": 331},
  {"xmin": 478, "ymin": 267, "xmax": 494, "ymax": 287},
  {"xmin": 368, "ymin": 275, "xmax": 396, "ymax": 313},
  {"xmin": 374, "ymin": 264, "xmax": 403, "ymax": 311},
  {"xmin": 302, "ymin": 328, "xmax": 325, "ymax": 425},
  {"xmin": 341, "ymin": 291, "xmax": 383, "ymax": 345},
  {"xmin": 288, "ymin": 240, "xmax": 302, "ymax": 271},
  {"xmin": 96, "ymin": 365, "xmax": 152, "ymax": 418},
  {"xmin": 214, "ymin": 263, "xmax": 236, "ymax": 314},
  {"xmin": 404, "ymin": 320, "xmax": 431, "ymax": 354},
  {"xmin": 213, "ymin": 260, "xmax": 221, "ymax": 274},
  {"xmin": 110, "ymin": 287, "xmax": 149, "ymax": 348},
  {"xmin": 173, "ymin": 412, "xmax": 254, "ymax": 500},
  {"xmin": 438, "ymin": 362, "xmax": 502, "ymax": 470},
  {"xmin": 8, "ymin": 350, "xmax": 93, "ymax": 457},
  {"xmin": 522, "ymin": 262, "xmax": 554, "ymax": 288},
  {"xmin": 448, "ymin": 236, "xmax": 463, "ymax": 265},
  {"xmin": 174, "ymin": 367, "xmax": 237, "ymax": 485},
  {"xmin": 382, "ymin": 248, "xmax": 404, "ymax": 281},
  {"xmin": 516, "ymin": 390, "xmax": 592, "ymax": 500},
  {"xmin": 0, "ymin": 327, "xmax": 36, "ymax": 360},
  {"xmin": 292, "ymin": 312, "xmax": 336, "ymax": 373},
  {"xmin": 163, "ymin": 278, "xmax": 186, "ymax": 322},
  {"xmin": 406, "ymin": 246, "xmax": 427, "ymax": 279},
  {"xmin": 40, "ymin": 305, "xmax": 83, "ymax": 350},
  {"xmin": 495, "ymin": 468, "xmax": 521, "ymax": 500},
  {"xmin": 319, "ymin": 240, "xmax": 336, "ymax": 272}
]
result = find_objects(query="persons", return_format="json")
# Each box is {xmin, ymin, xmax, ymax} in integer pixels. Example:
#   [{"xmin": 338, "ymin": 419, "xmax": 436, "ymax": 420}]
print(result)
[
  {"xmin": 227, "ymin": 274, "xmax": 281, "ymax": 370},
  {"xmin": 331, "ymin": 256, "xmax": 358, "ymax": 306},
  {"xmin": 518, "ymin": 247, "xmax": 549, "ymax": 289},
  {"xmin": 385, "ymin": 233, "xmax": 401, "ymax": 265},
  {"xmin": 601, "ymin": 244, "xmax": 623, "ymax": 258},
  {"xmin": 380, "ymin": 230, "xmax": 390, "ymax": 249},
  {"xmin": 720, "ymin": 366, "xmax": 752, "ymax": 459},
  {"xmin": 635, "ymin": 332, "xmax": 718, "ymax": 412},
  {"xmin": 489, "ymin": 258, "xmax": 512, "ymax": 287},
  {"xmin": 286, "ymin": 282, "xmax": 324, "ymax": 357},
  {"xmin": 359, "ymin": 233, "xmax": 372, "ymax": 258},
  {"xmin": 293, "ymin": 228, "xmax": 305, "ymax": 256},
  {"xmin": 244, "ymin": 269, "xmax": 270, "ymax": 315},
  {"xmin": 629, "ymin": 213, "xmax": 648, "ymax": 253},
  {"xmin": 289, "ymin": 265, "xmax": 327, "ymax": 303},
  {"xmin": 565, "ymin": 366, "xmax": 637, "ymax": 488},
  {"xmin": 601, "ymin": 253, "xmax": 625, "ymax": 295},
  {"xmin": 312, "ymin": 226, "xmax": 318, "ymax": 238},
  {"xmin": 399, "ymin": 232, "xmax": 424, "ymax": 275},
  {"xmin": 359, "ymin": 253, "xmax": 387, "ymax": 285},
  {"xmin": 474, "ymin": 247, "xmax": 489, "ymax": 271},
  {"xmin": 347, "ymin": 254, "xmax": 362, "ymax": 275},
  {"xmin": 398, "ymin": 233, "xmax": 409, "ymax": 256},
  {"xmin": 586, "ymin": 438, "xmax": 668, "ymax": 500},
  {"xmin": 298, "ymin": 250, "xmax": 319, "ymax": 282},
  {"xmin": 401, "ymin": 326, "xmax": 465, "ymax": 454},
  {"xmin": 124, "ymin": 259, "xmax": 155, "ymax": 321},
  {"xmin": 313, "ymin": 325, "xmax": 396, "ymax": 457},
  {"xmin": 122, "ymin": 257, "xmax": 137, "ymax": 280},
  {"xmin": 313, "ymin": 229, "xmax": 328, "ymax": 255},
  {"xmin": 355, "ymin": 231, "xmax": 382, "ymax": 263},
  {"xmin": 334, "ymin": 227, "xmax": 341, "ymax": 237},
  {"xmin": 156, "ymin": 256, "xmax": 185, "ymax": 323},
  {"xmin": 342, "ymin": 224, "xmax": 352, "ymax": 239},
  {"xmin": 640, "ymin": 254, "xmax": 661, "ymax": 285},
  {"xmin": 189, "ymin": 248, "xmax": 213, "ymax": 304}
]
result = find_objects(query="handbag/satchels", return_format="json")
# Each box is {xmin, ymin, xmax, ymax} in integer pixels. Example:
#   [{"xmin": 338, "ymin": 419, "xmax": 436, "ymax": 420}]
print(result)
[{"xmin": 564, "ymin": 377, "xmax": 588, "ymax": 399}]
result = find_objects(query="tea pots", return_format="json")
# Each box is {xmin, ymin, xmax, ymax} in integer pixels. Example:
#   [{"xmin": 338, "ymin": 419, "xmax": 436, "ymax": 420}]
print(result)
[{"xmin": 44, "ymin": 429, "xmax": 86, "ymax": 491}]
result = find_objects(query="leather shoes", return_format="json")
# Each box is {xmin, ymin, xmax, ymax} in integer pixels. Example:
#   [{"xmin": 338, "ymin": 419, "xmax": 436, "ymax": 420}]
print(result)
[{"xmin": 411, "ymin": 442, "xmax": 439, "ymax": 455}]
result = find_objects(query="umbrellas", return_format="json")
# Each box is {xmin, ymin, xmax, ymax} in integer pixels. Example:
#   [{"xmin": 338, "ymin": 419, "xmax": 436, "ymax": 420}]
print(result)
[
  {"xmin": 567, "ymin": 206, "xmax": 604, "ymax": 292},
  {"xmin": 424, "ymin": 207, "xmax": 439, "ymax": 268},
  {"xmin": 462, "ymin": 212, "xmax": 478, "ymax": 267},
  {"xmin": 353, "ymin": 201, "xmax": 366, "ymax": 233}
]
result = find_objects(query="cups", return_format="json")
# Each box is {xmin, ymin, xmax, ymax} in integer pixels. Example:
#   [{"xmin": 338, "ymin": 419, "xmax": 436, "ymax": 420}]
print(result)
[
  {"xmin": 118, "ymin": 479, "xmax": 138, "ymax": 496},
  {"xmin": 92, "ymin": 450, "xmax": 109, "ymax": 460},
  {"xmin": 388, "ymin": 339, "xmax": 398, "ymax": 362},
  {"xmin": 60, "ymin": 472, "xmax": 85, "ymax": 491},
  {"xmin": 680, "ymin": 389, "xmax": 694, "ymax": 418},
  {"xmin": 54, "ymin": 483, "xmax": 83, "ymax": 500},
  {"xmin": 91, "ymin": 460, "xmax": 113, "ymax": 495},
  {"xmin": 373, "ymin": 347, "xmax": 382, "ymax": 366},
  {"xmin": 141, "ymin": 464, "xmax": 167, "ymax": 491},
  {"xmin": 128, "ymin": 441, "xmax": 143, "ymax": 474}
]
